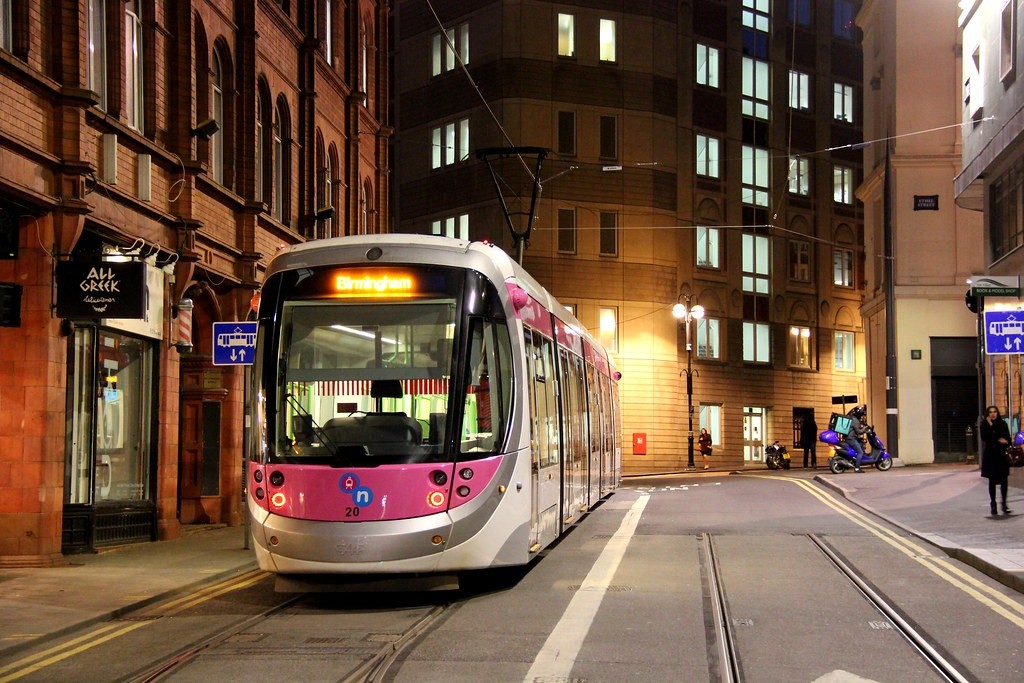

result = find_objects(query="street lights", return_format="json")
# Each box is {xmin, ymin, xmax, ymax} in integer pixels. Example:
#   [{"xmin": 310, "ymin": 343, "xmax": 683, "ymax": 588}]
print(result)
[{"xmin": 671, "ymin": 293, "xmax": 704, "ymax": 471}]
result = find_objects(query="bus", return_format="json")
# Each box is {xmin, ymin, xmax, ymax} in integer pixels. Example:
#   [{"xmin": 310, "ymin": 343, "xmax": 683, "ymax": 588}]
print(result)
[{"xmin": 248, "ymin": 234, "xmax": 623, "ymax": 591}]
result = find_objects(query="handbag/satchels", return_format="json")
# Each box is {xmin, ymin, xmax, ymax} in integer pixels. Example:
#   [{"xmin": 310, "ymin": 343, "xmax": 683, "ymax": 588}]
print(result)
[
  {"xmin": 1005, "ymin": 446, "xmax": 1024, "ymax": 467},
  {"xmin": 693, "ymin": 443, "xmax": 702, "ymax": 451}
]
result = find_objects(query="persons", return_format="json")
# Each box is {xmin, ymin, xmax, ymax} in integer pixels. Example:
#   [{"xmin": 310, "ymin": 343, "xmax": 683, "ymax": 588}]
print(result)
[
  {"xmin": 979, "ymin": 405, "xmax": 1013, "ymax": 517},
  {"xmin": 698, "ymin": 428, "xmax": 712, "ymax": 469},
  {"xmin": 844, "ymin": 407, "xmax": 868, "ymax": 473},
  {"xmin": 799, "ymin": 412, "xmax": 818, "ymax": 469}
]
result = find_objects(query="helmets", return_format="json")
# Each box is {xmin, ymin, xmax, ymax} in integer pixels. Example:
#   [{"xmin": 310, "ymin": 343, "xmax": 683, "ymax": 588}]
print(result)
[{"xmin": 852, "ymin": 407, "xmax": 864, "ymax": 419}]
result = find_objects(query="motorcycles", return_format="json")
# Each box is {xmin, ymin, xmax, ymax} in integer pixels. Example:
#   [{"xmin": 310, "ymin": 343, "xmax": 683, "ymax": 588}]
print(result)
[
  {"xmin": 819, "ymin": 424, "xmax": 893, "ymax": 473},
  {"xmin": 765, "ymin": 441, "xmax": 791, "ymax": 471}
]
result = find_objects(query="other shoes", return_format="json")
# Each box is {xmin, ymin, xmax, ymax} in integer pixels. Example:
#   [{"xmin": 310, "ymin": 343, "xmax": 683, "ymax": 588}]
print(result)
[
  {"xmin": 1002, "ymin": 502, "xmax": 1011, "ymax": 513},
  {"xmin": 854, "ymin": 468, "xmax": 865, "ymax": 473},
  {"xmin": 991, "ymin": 501, "xmax": 997, "ymax": 514}
]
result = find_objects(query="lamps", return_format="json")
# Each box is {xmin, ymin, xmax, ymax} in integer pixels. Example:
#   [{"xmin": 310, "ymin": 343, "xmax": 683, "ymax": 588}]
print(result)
[
  {"xmin": 315, "ymin": 206, "xmax": 335, "ymax": 221},
  {"xmin": 192, "ymin": 117, "xmax": 219, "ymax": 140}
]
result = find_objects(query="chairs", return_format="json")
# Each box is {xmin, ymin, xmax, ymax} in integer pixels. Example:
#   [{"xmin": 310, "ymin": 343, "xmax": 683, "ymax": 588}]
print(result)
[
  {"xmin": 436, "ymin": 338, "xmax": 453, "ymax": 365},
  {"xmin": 371, "ymin": 379, "xmax": 410, "ymax": 443}
]
